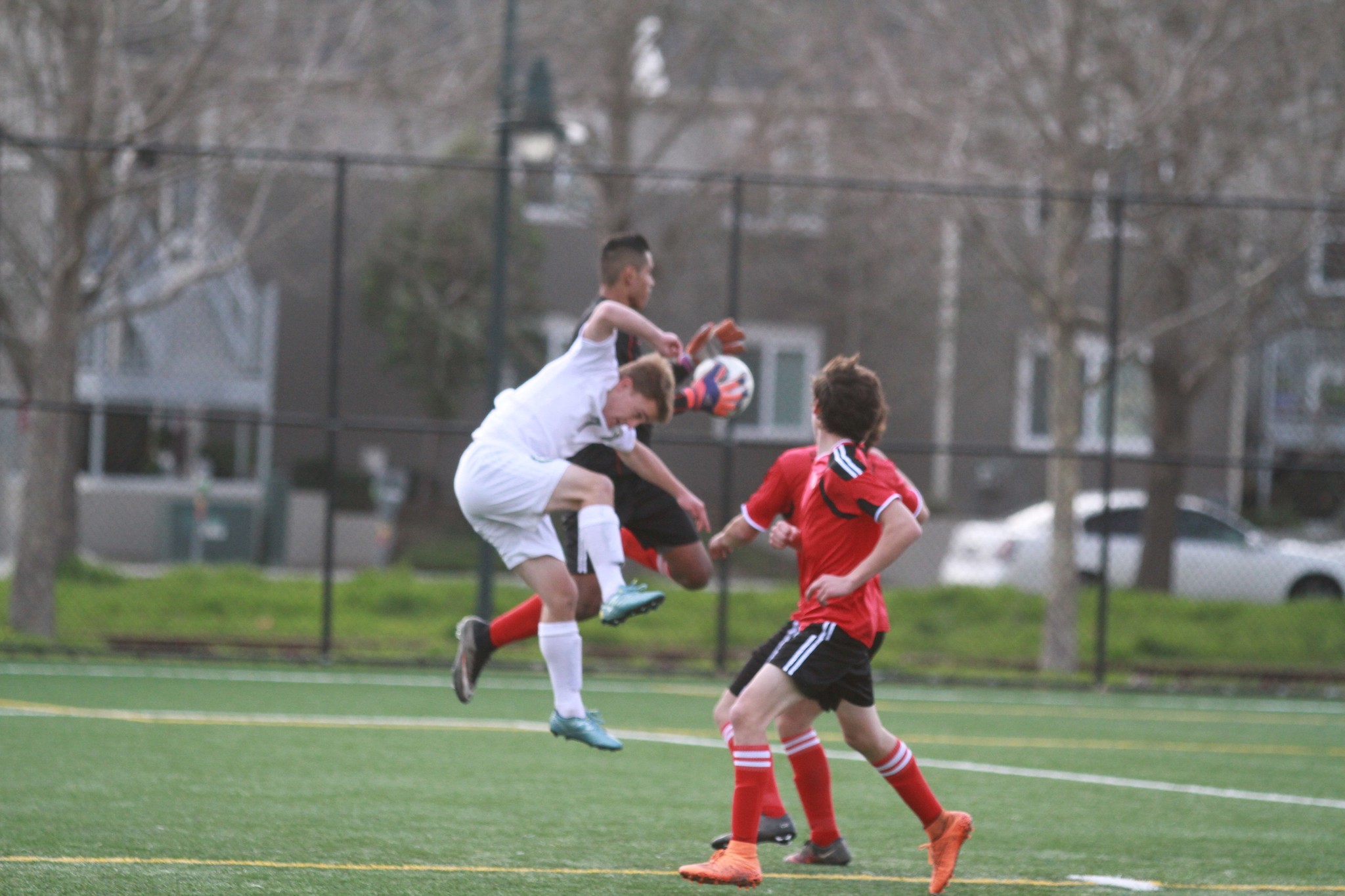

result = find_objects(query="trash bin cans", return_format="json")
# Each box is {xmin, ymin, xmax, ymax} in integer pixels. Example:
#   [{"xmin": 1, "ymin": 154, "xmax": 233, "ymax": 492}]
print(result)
[{"xmin": 167, "ymin": 504, "xmax": 252, "ymax": 564}]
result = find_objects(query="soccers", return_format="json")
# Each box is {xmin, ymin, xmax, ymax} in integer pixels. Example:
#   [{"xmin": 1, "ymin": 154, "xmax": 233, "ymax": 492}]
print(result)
[{"xmin": 692, "ymin": 354, "xmax": 755, "ymax": 422}]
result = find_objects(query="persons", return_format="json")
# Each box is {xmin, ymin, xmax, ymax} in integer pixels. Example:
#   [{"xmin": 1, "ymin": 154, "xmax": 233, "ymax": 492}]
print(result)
[
  {"xmin": 677, "ymin": 352, "xmax": 974, "ymax": 892},
  {"xmin": 709, "ymin": 400, "xmax": 928, "ymax": 868},
  {"xmin": 452, "ymin": 235, "xmax": 746, "ymax": 707},
  {"xmin": 454, "ymin": 300, "xmax": 709, "ymax": 752}
]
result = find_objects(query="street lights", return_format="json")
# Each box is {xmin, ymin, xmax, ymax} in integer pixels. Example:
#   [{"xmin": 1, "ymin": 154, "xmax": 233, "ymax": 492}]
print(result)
[{"xmin": 478, "ymin": 0, "xmax": 571, "ymax": 622}]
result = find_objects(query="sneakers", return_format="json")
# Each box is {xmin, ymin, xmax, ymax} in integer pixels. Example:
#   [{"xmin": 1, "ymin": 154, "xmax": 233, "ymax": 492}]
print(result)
[
  {"xmin": 452, "ymin": 615, "xmax": 498, "ymax": 704},
  {"xmin": 785, "ymin": 837, "xmax": 852, "ymax": 866},
  {"xmin": 918, "ymin": 811, "xmax": 974, "ymax": 893},
  {"xmin": 678, "ymin": 850, "xmax": 762, "ymax": 890},
  {"xmin": 711, "ymin": 814, "xmax": 797, "ymax": 850},
  {"xmin": 550, "ymin": 709, "xmax": 623, "ymax": 751},
  {"xmin": 600, "ymin": 583, "xmax": 665, "ymax": 626}
]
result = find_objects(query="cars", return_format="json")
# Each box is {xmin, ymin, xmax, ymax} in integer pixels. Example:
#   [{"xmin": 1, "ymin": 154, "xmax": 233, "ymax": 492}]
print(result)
[{"xmin": 935, "ymin": 485, "xmax": 1345, "ymax": 603}]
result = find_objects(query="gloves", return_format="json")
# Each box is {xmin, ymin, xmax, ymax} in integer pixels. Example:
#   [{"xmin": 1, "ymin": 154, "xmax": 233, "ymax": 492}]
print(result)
[
  {"xmin": 685, "ymin": 319, "xmax": 746, "ymax": 370},
  {"xmin": 684, "ymin": 363, "xmax": 748, "ymax": 415}
]
